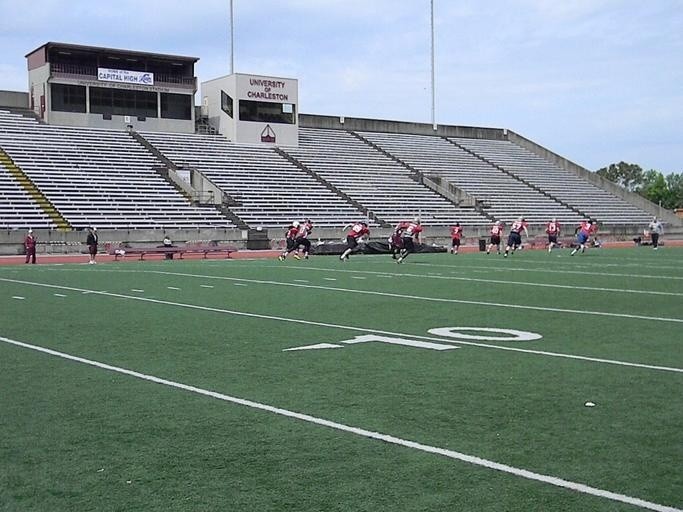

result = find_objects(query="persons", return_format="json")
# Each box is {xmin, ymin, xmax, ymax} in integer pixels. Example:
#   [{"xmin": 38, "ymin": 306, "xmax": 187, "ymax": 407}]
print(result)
[
  {"xmin": 451, "ymin": 224, "xmax": 462, "ymax": 254},
  {"xmin": 25, "ymin": 230, "xmax": 36, "ymax": 264},
  {"xmin": 164, "ymin": 236, "xmax": 172, "ymax": 247},
  {"xmin": 649, "ymin": 216, "xmax": 663, "ymax": 250},
  {"xmin": 278, "ymin": 220, "xmax": 312, "ymax": 261},
  {"xmin": 392, "ymin": 222, "xmax": 409, "ymax": 259},
  {"xmin": 570, "ymin": 221, "xmax": 593, "ymax": 256},
  {"xmin": 340, "ymin": 221, "xmax": 369, "ymax": 262},
  {"xmin": 487, "ymin": 221, "xmax": 502, "ymax": 254},
  {"xmin": 285, "ymin": 221, "xmax": 300, "ymax": 260},
  {"xmin": 545, "ymin": 219, "xmax": 560, "ymax": 252},
  {"xmin": 396, "ymin": 219, "xmax": 422, "ymax": 263},
  {"xmin": 504, "ymin": 217, "xmax": 528, "ymax": 257},
  {"xmin": 593, "ymin": 237, "xmax": 599, "ymax": 248},
  {"xmin": 87, "ymin": 227, "xmax": 98, "ymax": 264}
]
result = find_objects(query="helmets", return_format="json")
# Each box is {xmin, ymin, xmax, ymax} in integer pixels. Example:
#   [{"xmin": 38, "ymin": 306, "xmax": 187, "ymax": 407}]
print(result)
[
  {"xmin": 494, "ymin": 215, "xmax": 559, "ymax": 226},
  {"xmin": 412, "ymin": 218, "xmax": 421, "ymax": 225},
  {"xmin": 292, "ymin": 221, "xmax": 301, "ymax": 229}
]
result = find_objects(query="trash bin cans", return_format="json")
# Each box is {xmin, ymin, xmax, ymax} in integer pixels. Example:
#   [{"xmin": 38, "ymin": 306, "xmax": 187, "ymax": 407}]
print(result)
[{"xmin": 479, "ymin": 240, "xmax": 485, "ymax": 251}]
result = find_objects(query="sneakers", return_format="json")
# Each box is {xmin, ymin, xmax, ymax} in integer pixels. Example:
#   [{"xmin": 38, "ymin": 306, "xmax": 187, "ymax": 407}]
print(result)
[
  {"xmin": 486, "ymin": 244, "xmax": 591, "ymax": 259},
  {"xmin": 450, "ymin": 248, "xmax": 459, "ymax": 255},
  {"xmin": 277, "ymin": 253, "xmax": 310, "ymax": 263},
  {"xmin": 337, "ymin": 251, "xmax": 406, "ymax": 265}
]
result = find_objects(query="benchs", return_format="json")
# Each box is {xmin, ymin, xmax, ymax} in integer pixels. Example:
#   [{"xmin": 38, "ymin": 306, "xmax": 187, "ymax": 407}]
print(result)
[
  {"xmin": 0, "ymin": 110, "xmax": 672, "ymax": 231},
  {"xmin": 526, "ymin": 238, "xmax": 591, "ymax": 249},
  {"xmin": 108, "ymin": 243, "xmax": 240, "ymax": 262}
]
